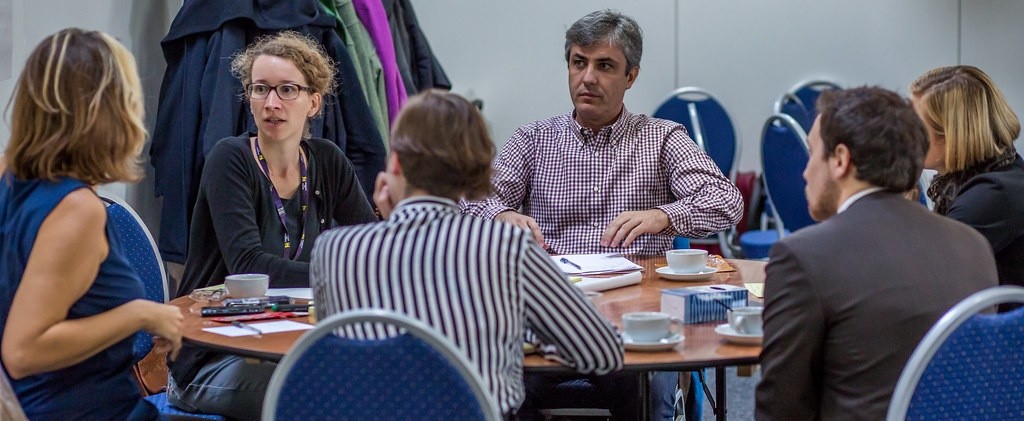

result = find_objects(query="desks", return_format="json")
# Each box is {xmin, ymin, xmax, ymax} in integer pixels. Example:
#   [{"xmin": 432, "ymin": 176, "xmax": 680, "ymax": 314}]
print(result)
[{"xmin": 165, "ymin": 257, "xmax": 769, "ymax": 421}]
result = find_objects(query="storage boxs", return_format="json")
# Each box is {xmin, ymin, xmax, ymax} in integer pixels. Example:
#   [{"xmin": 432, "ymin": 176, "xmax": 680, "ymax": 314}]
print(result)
[{"xmin": 658, "ymin": 284, "xmax": 749, "ymax": 324}]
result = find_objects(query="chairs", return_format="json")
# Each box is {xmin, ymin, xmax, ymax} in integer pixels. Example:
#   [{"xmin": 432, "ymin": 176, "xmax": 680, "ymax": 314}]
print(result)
[
  {"xmin": 261, "ymin": 308, "xmax": 501, "ymax": 420},
  {"xmin": 536, "ymin": 79, "xmax": 1024, "ymax": 421},
  {"xmin": 87, "ymin": 192, "xmax": 167, "ymax": 396}
]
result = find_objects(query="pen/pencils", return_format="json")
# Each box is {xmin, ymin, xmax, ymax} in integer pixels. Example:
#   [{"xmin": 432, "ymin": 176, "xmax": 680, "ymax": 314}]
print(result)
[
  {"xmin": 232, "ymin": 321, "xmax": 261, "ymax": 334},
  {"xmin": 560, "ymin": 257, "xmax": 581, "ymax": 270}
]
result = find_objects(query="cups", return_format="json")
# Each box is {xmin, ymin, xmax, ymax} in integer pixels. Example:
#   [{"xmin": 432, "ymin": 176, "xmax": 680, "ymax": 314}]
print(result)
[
  {"xmin": 727, "ymin": 306, "xmax": 765, "ymax": 335},
  {"xmin": 622, "ymin": 311, "xmax": 684, "ymax": 344},
  {"xmin": 225, "ymin": 273, "xmax": 270, "ymax": 299},
  {"xmin": 666, "ymin": 249, "xmax": 708, "ymax": 274}
]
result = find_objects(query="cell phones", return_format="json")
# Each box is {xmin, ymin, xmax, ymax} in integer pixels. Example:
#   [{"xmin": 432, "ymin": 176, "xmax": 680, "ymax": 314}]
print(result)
[
  {"xmin": 201, "ymin": 305, "xmax": 265, "ymax": 315},
  {"xmin": 278, "ymin": 305, "xmax": 313, "ymax": 312}
]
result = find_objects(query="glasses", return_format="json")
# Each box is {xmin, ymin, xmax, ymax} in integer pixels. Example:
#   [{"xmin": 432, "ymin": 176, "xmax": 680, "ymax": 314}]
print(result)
[
  {"xmin": 189, "ymin": 288, "xmax": 229, "ymax": 304},
  {"xmin": 244, "ymin": 81, "xmax": 315, "ymax": 101}
]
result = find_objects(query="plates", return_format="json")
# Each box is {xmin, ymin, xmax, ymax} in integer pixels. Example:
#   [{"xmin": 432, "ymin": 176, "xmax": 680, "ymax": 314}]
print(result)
[
  {"xmin": 713, "ymin": 323, "xmax": 764, "ymax": 343},
  {"xmin": 619, "ymin": 331, "xmax": 686, "ymax": 351},
  {"xmin": 655, "ymin": 266, "xmax": 719, "ymax": 281}
]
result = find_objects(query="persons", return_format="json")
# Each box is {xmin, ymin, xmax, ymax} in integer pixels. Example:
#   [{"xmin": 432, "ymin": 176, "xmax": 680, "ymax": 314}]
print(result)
[
  {"xmin": 458, "ymin": 10, "xmax": 744, "ymax": 255},
  {"xmin": 310, "ymin": 88, "xmax": 625, "ymax": 421},
  {"xmin": 0, "ymin": 27, "xmax": 184, "ymax": 421},
  {"xmin": 754, "ymin": 86, "xmax": 999, "ymax": 421},
  {"xmin": 166, "ymin": 31, "xmax": 381, "ymax": 421},
  {"xmin": 909, "ymin": 65, "xmax": 1024, "ymax": 314}
]
